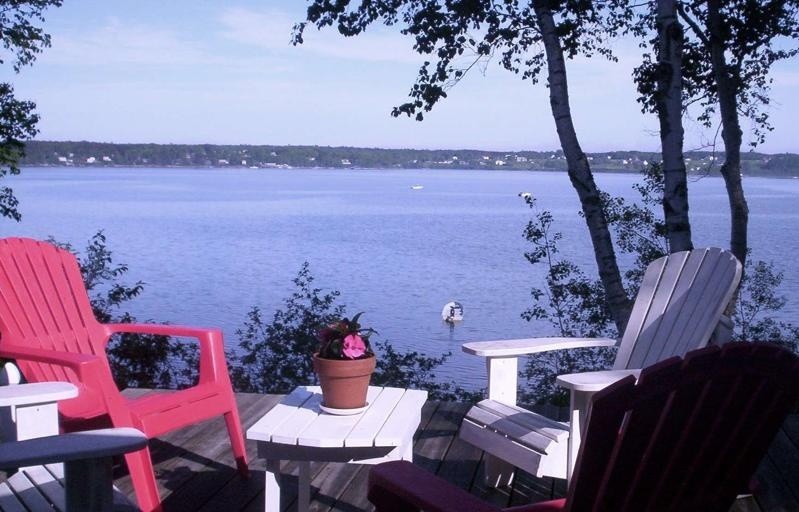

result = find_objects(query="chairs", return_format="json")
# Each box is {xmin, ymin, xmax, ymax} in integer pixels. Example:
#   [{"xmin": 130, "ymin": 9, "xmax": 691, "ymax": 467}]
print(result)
[
  {"xmin": 0, "ymin": 237, "xmax": 248, "ymax": 512},
  {"xmin": 458, "ymin": 247, "xmax": 742, "ymax": 498},
  {"xmin": 0, "ymin": 362, "xmax": 149, "ymax": 512},
  {"xmin": 371, "ymin": 339, "xmax": 797, "ymax": 512}
]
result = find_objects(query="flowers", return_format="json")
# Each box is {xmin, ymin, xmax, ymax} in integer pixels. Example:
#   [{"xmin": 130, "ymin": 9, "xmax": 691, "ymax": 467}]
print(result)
[{"xmin": 313, "ymin": 311, "xmax": 380, "ymax": 360}]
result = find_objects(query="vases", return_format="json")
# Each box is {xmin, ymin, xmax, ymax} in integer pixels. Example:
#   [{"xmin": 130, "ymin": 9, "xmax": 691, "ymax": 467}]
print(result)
[{"xmin": 312, "ymin": 352, "xmax": 376, "ymax": 415}]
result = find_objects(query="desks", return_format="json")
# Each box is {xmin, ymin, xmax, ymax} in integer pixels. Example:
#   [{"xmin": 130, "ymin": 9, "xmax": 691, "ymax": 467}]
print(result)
[{"xmin": 246, "ymin": 386, "xmax": 428, "ymax": 512}]
